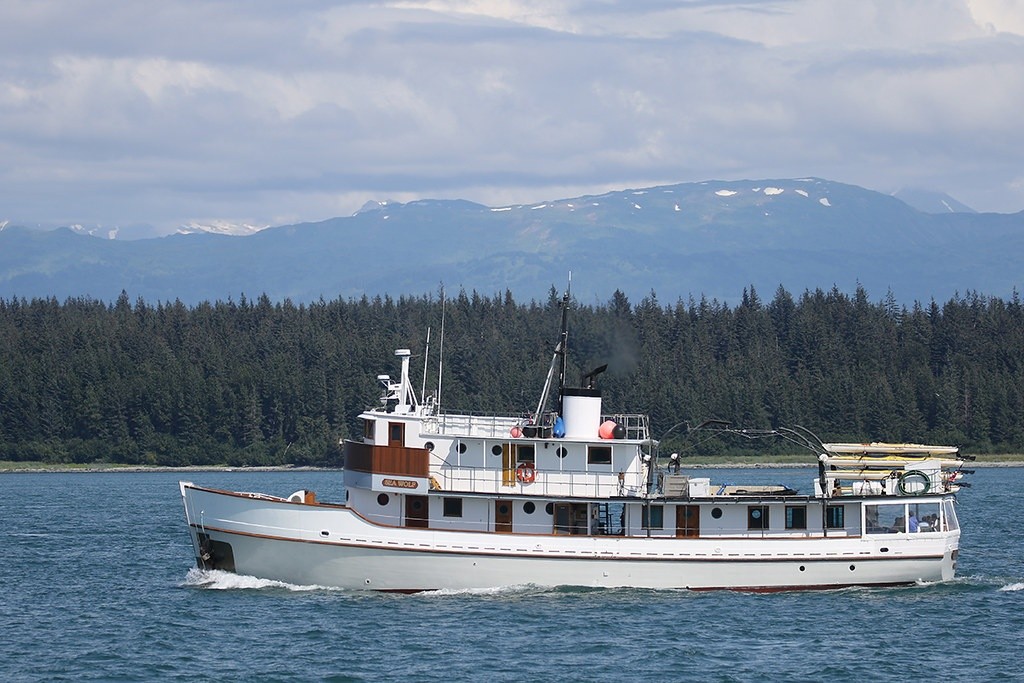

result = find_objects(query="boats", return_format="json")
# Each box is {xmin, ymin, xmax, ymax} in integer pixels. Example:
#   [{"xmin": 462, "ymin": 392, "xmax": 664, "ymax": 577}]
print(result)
[{"xmin": 176, "ymin": 267, "xmax": 979, "ymax": 596}]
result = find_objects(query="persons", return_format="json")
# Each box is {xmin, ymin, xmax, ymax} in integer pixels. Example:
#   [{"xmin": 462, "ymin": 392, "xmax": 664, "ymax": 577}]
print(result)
[{"xmin": 894, "ymin": 511, "xmax": 945, "ymax": 533}]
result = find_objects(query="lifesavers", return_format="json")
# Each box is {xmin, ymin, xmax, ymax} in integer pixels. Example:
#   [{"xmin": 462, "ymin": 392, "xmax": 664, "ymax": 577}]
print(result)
[{"xmin": 517, "ymin": 462, "xmax": 537, "ymax": 482}]
